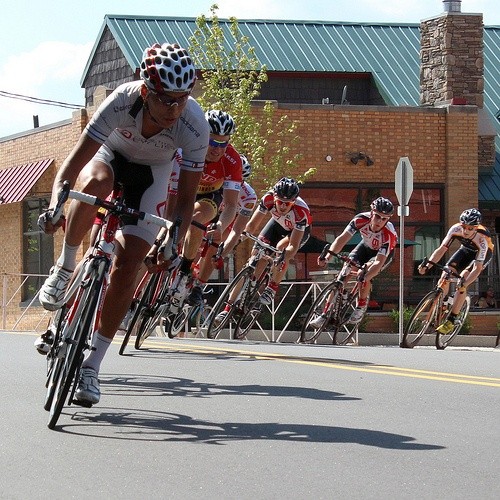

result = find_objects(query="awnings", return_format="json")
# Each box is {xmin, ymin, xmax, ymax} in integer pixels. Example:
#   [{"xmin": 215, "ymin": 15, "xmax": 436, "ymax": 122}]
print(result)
[{"xmin": 0, "ymin": 159, "xmax": 55, "ymax": 205}]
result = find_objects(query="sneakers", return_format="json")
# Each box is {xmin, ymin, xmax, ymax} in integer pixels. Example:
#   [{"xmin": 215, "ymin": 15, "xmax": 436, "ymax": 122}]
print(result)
[
  {"xmin": 436, "ymin": 319, "xmax": 454, "ymax": 335},
  {"xmin": 261, "ymin": 286, "xmax": 276, "ymax": 305},
  {"xmin": 39, "ymin": 264, "xmax": 75, "ymax": 311},
  {"xmin": 349, "ymin": 303, "xmax": 367, "ymax": 324},
  {"xmin": 34, "ymin": 323, "xmax": 58, "ymax": 354},
  {"xmin": 214, "ymin": 310, "xmax": 228, "ymax": 327},
  {"xmin": 75, "ymin": 365, "xmax": 101, "ymax": 403},
  {"xmin": 310, "ymin": 313, "xmax": 326, "ymax": 329}
]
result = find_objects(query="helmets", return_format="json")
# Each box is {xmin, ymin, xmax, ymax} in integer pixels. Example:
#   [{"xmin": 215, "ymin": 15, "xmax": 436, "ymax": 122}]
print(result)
[
  {"xmin": 273, "ymin": 177, "xmax": 299, "ymax": 202},
  {"xmin": 140, "ymin": 43, "xmax": 197, "ymax": 93},
  {"xmin": 370, "ymin": 197, "xmax": 394, "ymax": 216},
  {"xmin": 239, "ymin": 153, "xmax": 250, "ymax": 181},
  {"xmin": 205, "ymin": 109, "xmax": 235, "ymax": 136},
  {"xmin": 460, "ymin": 208, "xmax": 482, "ymax": 225}
]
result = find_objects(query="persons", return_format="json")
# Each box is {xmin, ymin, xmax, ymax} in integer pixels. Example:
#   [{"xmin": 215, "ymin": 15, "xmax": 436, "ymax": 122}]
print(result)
[
  {"xmin": 37, "ymin": 40, "xmax": 211, "ymax": 405},
  {"xmin": 309, "ymin": 197, "xmax": 399, "ymax": 329},
  {"xmin": 417, "ymin": 209, "xmax": 495, "ymax": 335},
  {"xmin": 119, "ymin": 153, "xmax": 257, "ymax": 330},
  {"xmin": 212, "ymin": 177, "xmax": 312, "ymax": 328},
  {"xmin": 86, "ymin": 108, "xmax": 242, "ymax": 300}
]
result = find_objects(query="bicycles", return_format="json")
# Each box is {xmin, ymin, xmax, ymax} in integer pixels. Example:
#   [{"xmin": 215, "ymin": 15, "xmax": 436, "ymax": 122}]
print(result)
[
  {"xmin": 207, "ymin": 232, "xmax": 287, "ymax": 340},
  {"xmin": 36, "ymin": 179, "xmax": 184, "ymax": 430},
  {"xmin": 401, "ymin": 256, "xmax": 471, "ymax": 350},
  {"xmin": 299, "ymin": 243, "xmax": 374, "ymax": 346},
  {"xmin": 164, "ymin": 223, "xmax": 225, "ymax": 340},
  {"xmin": 118, "ymin": 219, "xmax": 218, "ymax": 356}
]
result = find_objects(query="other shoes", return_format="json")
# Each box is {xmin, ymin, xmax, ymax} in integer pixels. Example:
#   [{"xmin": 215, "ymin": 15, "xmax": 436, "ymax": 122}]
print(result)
[
  {"xmin": 169, "ymin": 271, "xmax": 187, "ymax": 297},
  {"xmin": 188, "ymin": 285, "xmax": 202, "ymax": 307},
  {"xmin": 117, "ymin": 312, "xmax": 132, "ymax": 330}
]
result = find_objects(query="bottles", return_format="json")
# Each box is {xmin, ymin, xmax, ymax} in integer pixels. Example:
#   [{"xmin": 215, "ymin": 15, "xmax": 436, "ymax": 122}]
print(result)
[
  {"xmin": 441, "ymin": 295, "xmax": 454, "ymax": 315},
  {"xmin": 250, "ymin": 276, "xmax": 256, "ymax": 294},
  {"xmin": 341, "ymin": 290, "xmax": 348, "ymax": 309},
  {"xmin": 175, "ymin": 274, "xmax": 188, "ymax": 298}
]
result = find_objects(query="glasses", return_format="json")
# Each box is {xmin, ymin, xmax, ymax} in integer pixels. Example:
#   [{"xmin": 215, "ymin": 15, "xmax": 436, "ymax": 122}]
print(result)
[
  {"xmin": 373, "ymin": 212, "xmax": 390, "ymax": 221},
  {"xmin": 209, "ymin": 138, "xmax": 231, "ymax": 148},
  {"xmin": 276, "ymin": 199, "xmax": 293, "ymax": 206},
  {"xmin": 144, "ymin": 82, "xmax": 190, "ymax": 109},
  {"xmin": 461, "ymin": 225, "xmax": 475, "ymax": 231}
]
